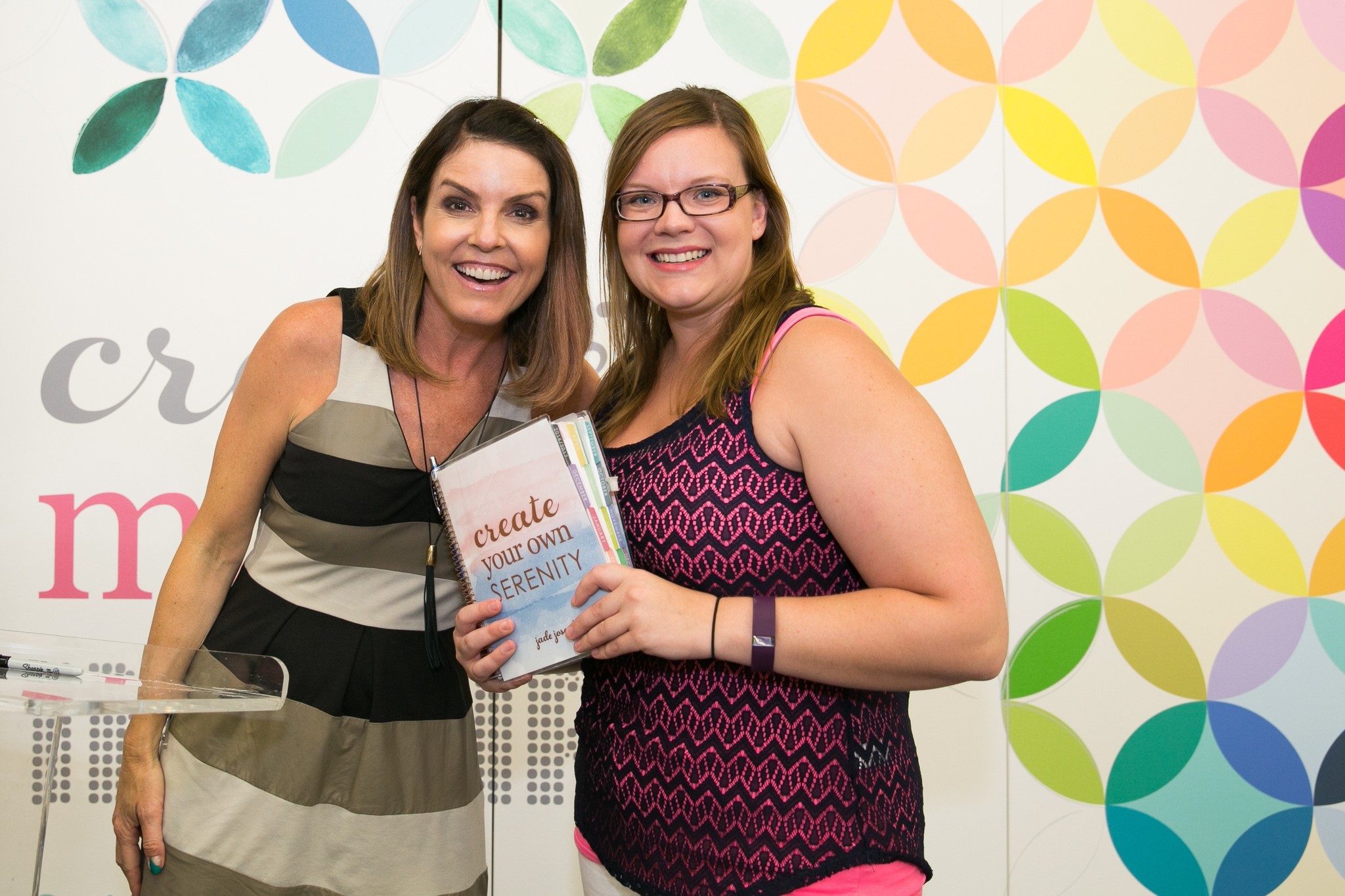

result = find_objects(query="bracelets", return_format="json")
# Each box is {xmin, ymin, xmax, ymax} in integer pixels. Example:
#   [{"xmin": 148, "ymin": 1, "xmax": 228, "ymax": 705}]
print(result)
[
  {"xmin": 711, "ymin": 597, "xmax": 720, "ymax": 658},
  {"xmin": 751, "ymin": 596, "xmax": 775, "ymax": 673}
]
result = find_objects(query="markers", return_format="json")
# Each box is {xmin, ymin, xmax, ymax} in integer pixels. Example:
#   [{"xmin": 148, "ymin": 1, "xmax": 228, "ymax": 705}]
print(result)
[{"xmin": 0, "ymin": 654, "xmax": 84, "ymax": 676}]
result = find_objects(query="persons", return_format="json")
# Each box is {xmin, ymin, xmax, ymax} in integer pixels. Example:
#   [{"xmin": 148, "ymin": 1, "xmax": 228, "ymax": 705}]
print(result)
[
  {"xmin": 113, "ymin": 98, "xmax": 598, "ymax": 896},
  {"xmin": 452, "ymin": 84, "xmax": 1008, "ymax": 896}
]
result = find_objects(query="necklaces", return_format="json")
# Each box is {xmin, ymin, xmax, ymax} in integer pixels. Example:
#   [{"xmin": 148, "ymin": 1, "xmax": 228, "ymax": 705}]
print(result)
[{"xmin": 415, "ymin": 348, "xmax": 509, "ymax": 671}]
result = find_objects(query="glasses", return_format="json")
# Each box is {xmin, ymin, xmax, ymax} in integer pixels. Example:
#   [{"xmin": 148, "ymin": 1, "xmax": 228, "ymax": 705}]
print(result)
[{"xmin": 611, "ymin": 183, "xmax": 754, "ymax": 222}]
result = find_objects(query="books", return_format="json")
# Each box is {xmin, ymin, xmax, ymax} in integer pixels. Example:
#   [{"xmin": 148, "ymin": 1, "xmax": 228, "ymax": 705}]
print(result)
[{"xmin": 432, "ymin": 413, "xmax": 634, "ymax": 686}]
